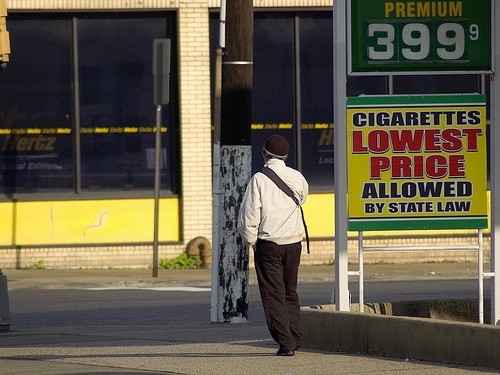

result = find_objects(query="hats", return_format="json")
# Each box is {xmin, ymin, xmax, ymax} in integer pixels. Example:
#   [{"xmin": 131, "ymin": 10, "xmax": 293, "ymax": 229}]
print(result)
[{"xmin": 262, "ymin": 134, "xmax": 290, "ymax": 157}]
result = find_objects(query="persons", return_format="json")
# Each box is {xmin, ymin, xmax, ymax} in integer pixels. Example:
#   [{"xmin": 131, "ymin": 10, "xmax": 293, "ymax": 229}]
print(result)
[{"xmin": 238, "ymin": 133, "xmax": 308, "ymax": 357}]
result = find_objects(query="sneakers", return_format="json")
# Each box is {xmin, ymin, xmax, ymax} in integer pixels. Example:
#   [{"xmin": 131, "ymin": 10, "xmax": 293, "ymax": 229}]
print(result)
[{"xmin": 276, "ymin": 349, "xmax": 294, "ymax": 356}]
